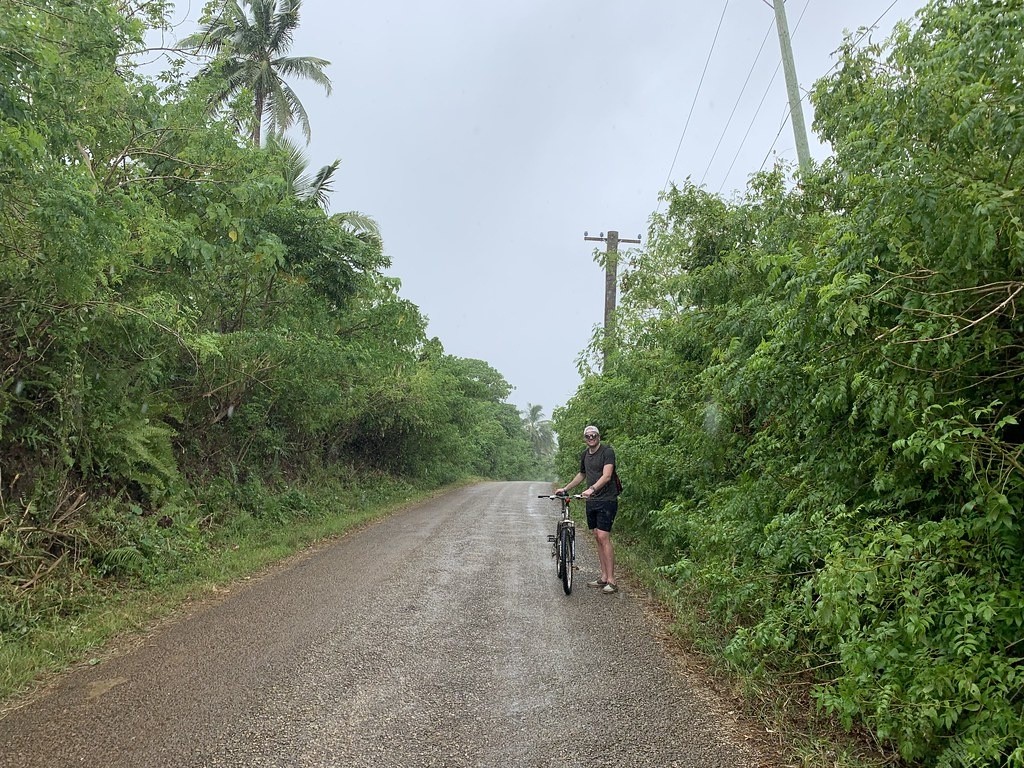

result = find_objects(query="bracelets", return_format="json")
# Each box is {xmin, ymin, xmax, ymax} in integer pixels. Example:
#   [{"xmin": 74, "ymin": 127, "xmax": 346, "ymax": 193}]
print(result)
[{"xmin": 589, "ymin": 485, "xmax": 596, "ymax": 492}]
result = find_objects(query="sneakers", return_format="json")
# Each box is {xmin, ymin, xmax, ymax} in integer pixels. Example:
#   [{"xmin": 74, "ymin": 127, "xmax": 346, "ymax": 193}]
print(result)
[
  {"xmin": 602, "ymin": 582, "xmax": 618, "ymax": 594},
  {"xmin": 587, "ymin": 578, "xmax": 607, "ymax": 588}
]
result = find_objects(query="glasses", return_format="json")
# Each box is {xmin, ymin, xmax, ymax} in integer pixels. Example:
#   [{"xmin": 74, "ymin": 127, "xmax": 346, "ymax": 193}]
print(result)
[{"xmin": 584, "ymin": 433, "xmax": 598, "ymax": 439}]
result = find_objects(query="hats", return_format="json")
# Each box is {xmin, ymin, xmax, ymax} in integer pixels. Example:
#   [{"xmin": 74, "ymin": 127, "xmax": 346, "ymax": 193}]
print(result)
[{"xmin": 583, "ymin": 426, "xmax": 599, "ymax": 435}]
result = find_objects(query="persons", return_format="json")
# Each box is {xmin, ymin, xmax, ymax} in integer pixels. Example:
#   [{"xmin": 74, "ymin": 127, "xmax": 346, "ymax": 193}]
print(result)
[{"xmin": 554, "ymin": 425, "xmax": 619, "ymax": 594}]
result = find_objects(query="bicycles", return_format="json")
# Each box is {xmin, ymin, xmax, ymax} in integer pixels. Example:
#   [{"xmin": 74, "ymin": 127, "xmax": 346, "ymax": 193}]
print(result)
[{"xmin": 538, "ymin": 490, "xmax": 589, "ymax": 595}]
